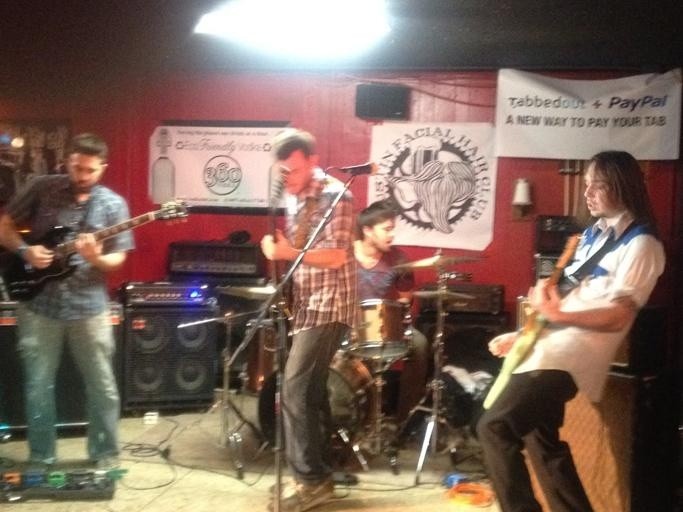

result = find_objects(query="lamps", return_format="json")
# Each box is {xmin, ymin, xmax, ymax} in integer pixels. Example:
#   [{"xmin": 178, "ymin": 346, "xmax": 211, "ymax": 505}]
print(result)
[{"xmin": 509, "ymin": 179, "xmax": 535, "ymax": 219}]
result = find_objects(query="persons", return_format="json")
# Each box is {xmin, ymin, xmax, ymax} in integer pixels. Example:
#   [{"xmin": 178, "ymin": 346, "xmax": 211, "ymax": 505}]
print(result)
[
  {"xmin": 1, "ymin": 131, "xmax": 134, "ymax": 472},
  {"xmin": 476, "ymin": 150, "xmax": 666, "ymax": 512},
  {"xmin": 261, "ymin": 130, "xmax": 357, "ymax": 512},
  {"xmin": 335, "ymin": 203, "xmax": 429, "ymax": 443}
]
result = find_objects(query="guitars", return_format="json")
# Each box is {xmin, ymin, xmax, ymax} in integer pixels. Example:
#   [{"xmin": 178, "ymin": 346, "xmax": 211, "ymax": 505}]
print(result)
[
  {"xmin": 482, "ymin": 235, "xmax": 580, "ymax": 408},
  {"xmin": 2, "ymin": 199, "xmax": 189, "ymax": 302},
  {"xmin": 268, "ymin": 166, "xmax": 293, "ymax": 320}
]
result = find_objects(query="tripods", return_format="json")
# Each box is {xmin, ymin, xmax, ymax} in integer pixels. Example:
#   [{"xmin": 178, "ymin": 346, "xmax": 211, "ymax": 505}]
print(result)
[
  {"xmin": 389, "ymin": 273, "xmax": 488, "ymax": 489},
  {"xmin": 245, "ymin": 301, "xmax": 416, "ymax": 484},
  {"xmin": 266, "ymin": 294, "xmax": 305, "ymax": 512},
  {"xmin": 167, "ymin": 310, "xmax": 266, "ymax": 481}
]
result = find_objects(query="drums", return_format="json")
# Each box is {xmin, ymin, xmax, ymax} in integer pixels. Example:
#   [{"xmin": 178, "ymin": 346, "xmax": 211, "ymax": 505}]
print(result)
[
  {"xmin": 258, "ymin": 352, "xmax": 375, "ymax": 456},
  {"xmin": 349, "ymin": 299, "xmax": 415, "ymax": 361}
]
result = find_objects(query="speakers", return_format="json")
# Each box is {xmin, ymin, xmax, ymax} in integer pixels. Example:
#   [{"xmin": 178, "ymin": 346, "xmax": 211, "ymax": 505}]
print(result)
[
  {"xmin": 2, "ymin": 312, "xmax": 122, "ymax": 430},
  {"xmin": 354, "ymin": 84, "xmax": 409, "ymax": 119},
  {"xmin": 121, "ymin": 282, "xmax": 215, "ymax": 416}
]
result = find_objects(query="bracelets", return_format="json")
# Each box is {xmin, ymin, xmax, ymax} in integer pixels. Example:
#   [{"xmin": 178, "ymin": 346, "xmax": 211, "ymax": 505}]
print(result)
[{"xmin": 16, "ymin": 243, "xmax": 28, "ymax": 257}]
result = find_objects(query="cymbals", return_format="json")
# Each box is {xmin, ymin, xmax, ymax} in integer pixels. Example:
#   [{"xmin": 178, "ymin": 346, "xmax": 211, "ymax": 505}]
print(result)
[
  {"xmin": 213, "ymin": 285, "xmax": 276, "ymax": 299},
  {"xmin": 394, "ymin": 256, "xmax": 484, "ymax": 268},
  {"xmin": 414, "ymin": 290, "xmax": 474, "ymax": 300}
]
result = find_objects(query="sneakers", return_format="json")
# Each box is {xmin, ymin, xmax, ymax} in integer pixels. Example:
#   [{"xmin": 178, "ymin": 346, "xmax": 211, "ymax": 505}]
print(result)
[
  {"xmin": 267, "ymin": 474, "xmax": 335, "ymax": 512},
  {"xmin": 28, "ymin": 459, "xmax": 55, "ymax": 469},
  {"xmin": 92, "ymin": 459, "xmax": 118, "ymax": 468}
]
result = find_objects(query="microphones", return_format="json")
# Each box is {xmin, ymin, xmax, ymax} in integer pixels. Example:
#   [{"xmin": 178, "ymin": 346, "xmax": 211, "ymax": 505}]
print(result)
[{"xmin": 339, "ymin": 162, "xmax": 377, "ymax": 176}]
